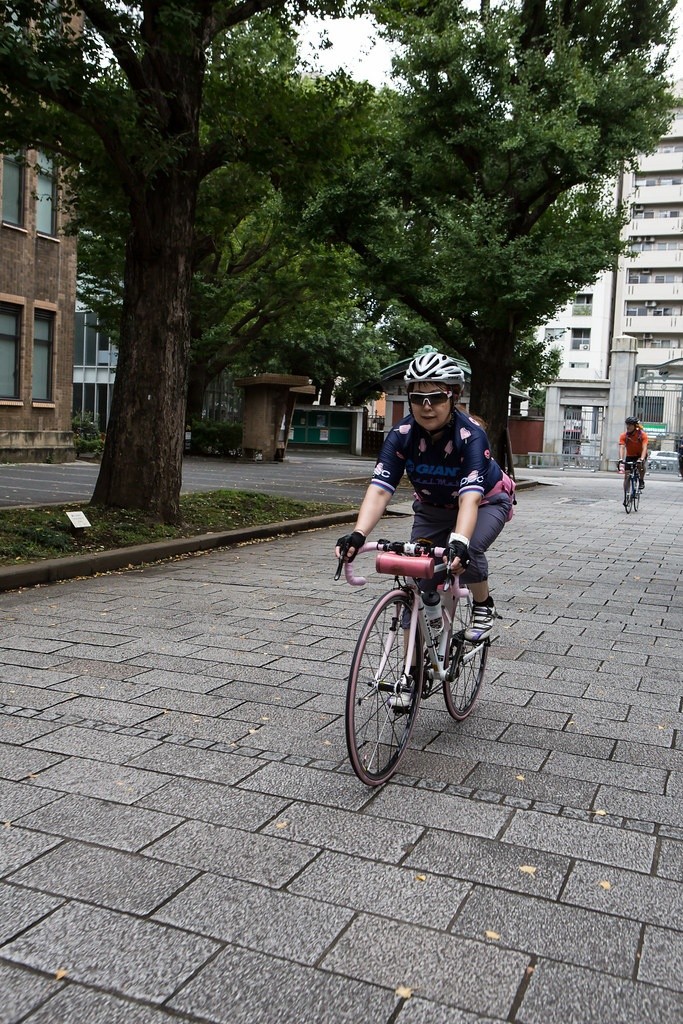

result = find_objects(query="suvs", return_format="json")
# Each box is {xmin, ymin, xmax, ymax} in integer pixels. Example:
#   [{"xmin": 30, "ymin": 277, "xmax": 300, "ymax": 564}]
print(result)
[{"xmin": 647, "ymin": 450, "xmax": 679, "ymax": 470}]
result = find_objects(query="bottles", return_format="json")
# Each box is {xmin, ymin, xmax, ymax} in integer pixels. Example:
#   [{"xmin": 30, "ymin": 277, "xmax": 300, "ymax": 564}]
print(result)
[{"xmin": 422, "ymin": 589, "xmax": 445, "ymax": 634}]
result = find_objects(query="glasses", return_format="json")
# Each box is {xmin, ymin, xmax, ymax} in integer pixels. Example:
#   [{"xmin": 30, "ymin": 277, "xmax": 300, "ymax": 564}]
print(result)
[{"xmin": 408, "ymin": 390, "xmax": 453, "ymax": 406}]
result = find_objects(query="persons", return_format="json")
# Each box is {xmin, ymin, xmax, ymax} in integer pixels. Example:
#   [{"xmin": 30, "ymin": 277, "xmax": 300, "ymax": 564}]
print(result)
[
  {"xmin": 618, "ymin": 415, "xmax": 650, "ymax": 507},
  {"xmin": 678, "ymin": 442, "xmax": 683, "ymax": 479},
  {"xmin": 335, "ymin": 353, "xmax": 517, "ymax": 706}
]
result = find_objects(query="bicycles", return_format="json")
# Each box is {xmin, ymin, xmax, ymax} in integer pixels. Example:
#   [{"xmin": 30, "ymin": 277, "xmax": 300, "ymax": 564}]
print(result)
[
  {"xmin": 334, "ymin": 538, "xmax": 503, "ymax": 786},
  {"xmin": 617, "ymin": 457, "xmax": 645, "ymax": 514}
]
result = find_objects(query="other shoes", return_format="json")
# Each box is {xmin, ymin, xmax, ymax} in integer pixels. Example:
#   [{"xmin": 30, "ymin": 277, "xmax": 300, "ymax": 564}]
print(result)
[
  {"xmin": 639, "ymin": 482, "xmax": 645, "ymax": 489},
  {"xmin": 623, "ymin": 499, "xmax": 628, "ymax": 505}
]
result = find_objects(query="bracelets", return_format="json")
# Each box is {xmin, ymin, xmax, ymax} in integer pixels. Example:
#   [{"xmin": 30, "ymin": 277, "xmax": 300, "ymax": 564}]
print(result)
[
  {"xmin": 619, "ymin": 459, "xmax": 623, "ymax": 461},
  {"xmin": 354, "ymin": 529, "xmax": 367, "ymax": 536}
]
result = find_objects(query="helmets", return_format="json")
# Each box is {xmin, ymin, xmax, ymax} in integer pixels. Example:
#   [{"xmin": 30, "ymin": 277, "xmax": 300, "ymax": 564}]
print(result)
[
  {"xmin": 404, "ymin": 351, "xmax": 464, "ymax": 398},
  {"xmin": 626, "ymin": 416, "xmax": 640, "ymax": 425}
]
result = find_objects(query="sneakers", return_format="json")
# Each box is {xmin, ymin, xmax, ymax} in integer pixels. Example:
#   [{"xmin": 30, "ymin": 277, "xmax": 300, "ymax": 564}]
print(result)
[
  {"xmin": 387, "ymin": 672, "xmax": 415, "ymax": 707},
  {"xmin": 464, "ymin": 596, "xmax": 503, "ymax": 642}
]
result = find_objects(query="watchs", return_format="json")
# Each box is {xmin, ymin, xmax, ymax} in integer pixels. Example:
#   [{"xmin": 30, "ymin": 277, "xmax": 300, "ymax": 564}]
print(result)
[{"xmin": 448, "ymin": 532, "xmax": 470, "ymax": 549}]
